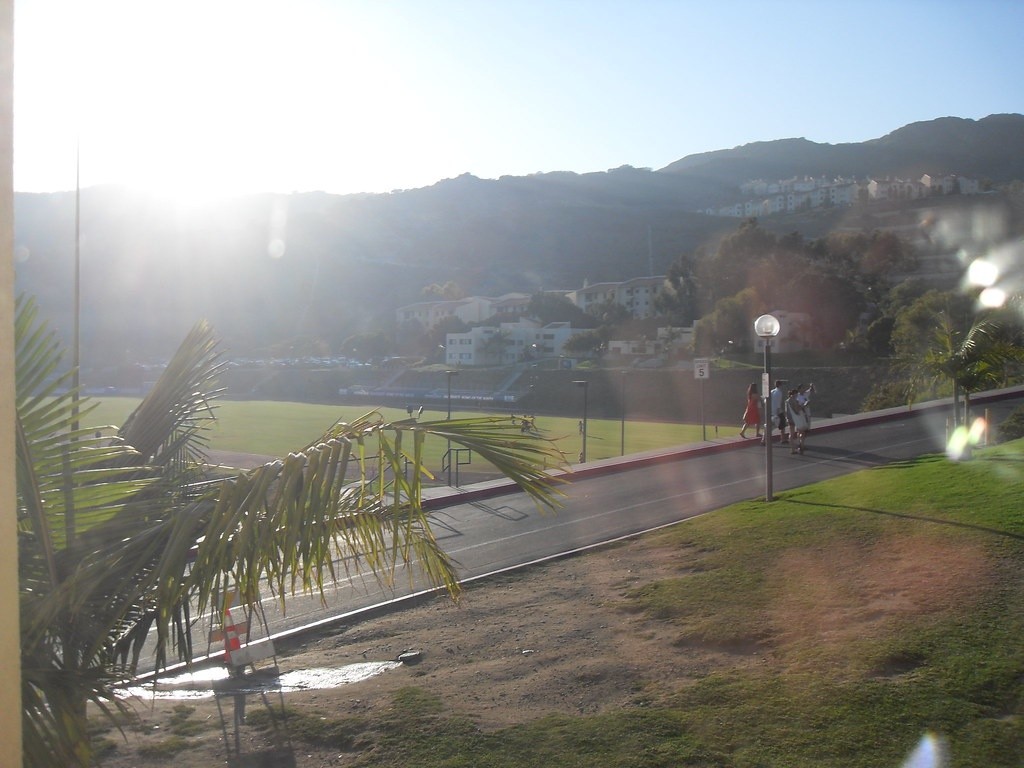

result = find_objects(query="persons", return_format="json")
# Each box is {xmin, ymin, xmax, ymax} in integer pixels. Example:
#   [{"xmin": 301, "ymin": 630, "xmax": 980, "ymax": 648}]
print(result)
[
  {"xmin": 576, "ymin": 421, "xmax": 583, "ymax": 435},
  {"xmin": 509, "ymin": 413, "xmax": 537, "ymax": 433},
  {"xmin": 740, "ymin": 380, "xmax": 815, "ymax": 455},
  {"xmin": 578, "ymin": 452, "xmax": 583, "ymax": 463}
]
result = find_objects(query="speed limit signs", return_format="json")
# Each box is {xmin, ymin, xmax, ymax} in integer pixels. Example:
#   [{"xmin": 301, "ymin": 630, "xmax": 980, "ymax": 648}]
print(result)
[{"xmin": 695, "ymin": 358, "xmax": 710, "ymax": 379}]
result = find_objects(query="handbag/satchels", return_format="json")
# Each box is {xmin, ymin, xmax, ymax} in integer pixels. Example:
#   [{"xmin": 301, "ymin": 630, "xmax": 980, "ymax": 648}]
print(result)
[{"xmin": 787, "ymin": 402, "xmax": 808, "ymax": 431}]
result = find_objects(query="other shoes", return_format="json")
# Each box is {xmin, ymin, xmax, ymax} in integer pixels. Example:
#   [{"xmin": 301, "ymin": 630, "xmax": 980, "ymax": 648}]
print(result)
[
  {"xmin": 760, "ymin": 440, "xmax": 765, "ymax": 446},
  {"xmin": 756, "ymin": 434, "xmax": 763, "ymax": 437},
  {"xmin": 781, "ymin": 440, "xmax": 789, "ymax": 443},
  {"xmin": 798, "ymin": 447, "xmax": 803, "ymax": 454},
  {"xmin": 740, "ymin": 432, "xmax": 745, "ymax": 437},
  {"xmin": 791, "ymin": 451, "xmax": 798, "ymax": 454}
]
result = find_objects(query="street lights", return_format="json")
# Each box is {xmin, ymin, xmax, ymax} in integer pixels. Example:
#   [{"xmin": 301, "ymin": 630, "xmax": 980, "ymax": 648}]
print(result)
[
  {"xmin": 755, "ymin": 314, "xmax": 780, "ymax": 502},
  {"xmin": 620, "ymin": 369, "xmax": 629, "ymax": 456},
  {"xmin": 445, "ymin": 370, "xmax": 459, "ymax": 450},
  {"xmin": 573, "ymin": 380, "xmax": 588, "ymax": 464}
]
